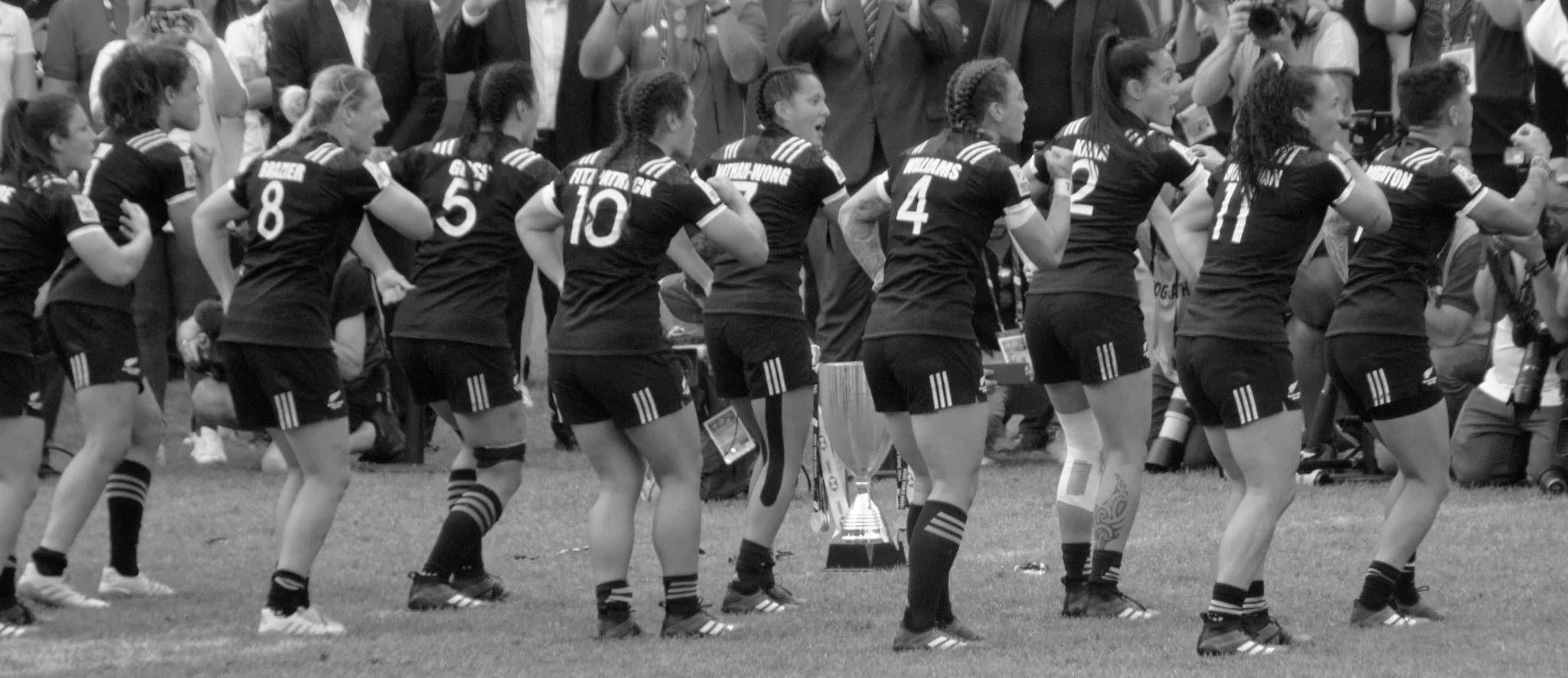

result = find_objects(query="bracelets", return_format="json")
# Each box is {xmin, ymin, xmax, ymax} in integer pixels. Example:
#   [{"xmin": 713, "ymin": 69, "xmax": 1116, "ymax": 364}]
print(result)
[
  {"xmin": 1344, "ymin": 158, "xmax": 1356, "ymax": 164},
  {"xmin": 178, "ymin": 339, "xmax": 193, "ymax": 353},
  {"xmin": 609, "ymin": 0, "xmax": 627, "ymax": 16},
  {"xmin": 1052, "ymin": 178, "xmax": 1072, "ymax": 198},
  {"xmin": 1530, "ymin": 157, "xmax": 1553, "ymax": 178},
  {"xmin": 709, "ymin": 5, "xmax": 732, "ymax": 20}
]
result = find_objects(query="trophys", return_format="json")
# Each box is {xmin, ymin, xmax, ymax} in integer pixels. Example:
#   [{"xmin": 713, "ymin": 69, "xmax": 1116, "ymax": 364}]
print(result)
[{"xmin": 817, "ymin": 362, "xmax": 906, "ymax": 571}]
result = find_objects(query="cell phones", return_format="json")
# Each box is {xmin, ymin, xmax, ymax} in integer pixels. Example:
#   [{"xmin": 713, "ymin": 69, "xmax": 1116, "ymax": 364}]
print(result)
[{"xmin": 149, "ymin": 10, "xmax": 196, "ymax": 34}]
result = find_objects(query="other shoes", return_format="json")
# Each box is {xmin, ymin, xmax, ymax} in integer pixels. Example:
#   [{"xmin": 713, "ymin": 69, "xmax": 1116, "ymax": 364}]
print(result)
[
  {"xmin": 999, "ymin": 430, "xmax": 1048, "ymax": 454},
  {"xmin": 0, "ymin": 617, "xmax": 42, "ymax": 635},
  {"xmin": 185, "ymin": 426, "xmax": 227, "ymax": 464},
  {"xmin": 262, "ymin": 440, "xmax": 289, "ymax": 476}
]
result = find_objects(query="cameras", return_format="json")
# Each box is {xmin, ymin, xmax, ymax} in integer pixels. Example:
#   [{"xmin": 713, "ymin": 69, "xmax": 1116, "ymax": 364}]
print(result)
[{"xmin": 1248, "ymin": 0, "xmax": 1290, "ymax": 39}]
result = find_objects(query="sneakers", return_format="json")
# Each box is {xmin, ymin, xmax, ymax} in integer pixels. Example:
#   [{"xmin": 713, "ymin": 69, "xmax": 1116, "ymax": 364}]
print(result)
[
  {"xmin": 1348, "ymin": 596, "xmax": 1430, "ymax": 628},
  {"xmin": 17, "ymin": 564, "xmax": 112, "ymax": 609},
  {"xmin": 1197, "ymin": 613, "xmax": 1290, "ymax": 654},
  {"xmin": 453, "ymin": 572, "xmax": 516, "ymax": 604},
  {"xmin": 99, "ymin": 566, "xmax": 174, "ymax": 597},
  {"xmin": 256, "ymin": 601, "xmax": 346, "ymax": 638},
  {"xmin": 407, "ymin": 570, "xmax": 494, "ymax": 610},
  {"xmin": 766, "ymin": 581, "xmax": 812, "ymax": 610},
  {"xmin": 600, "ymin": 609, "xmax": 650, "ymax": 638},
  {"xmin": 722, "ymin": 582, "xmax": 802, "ymax": 615},
  {"xmin": 1085, "ymin": 581, "xmax": 1156, "ymax": 621},
  {"xmin": 660, "ymin": 596, "xmax": 745, "ymax": 639},
  {"xmin": 1235, "ymin": 610, "xmax": 1313, "ymax": 646},
  {"xmin": 1062, "ymin": 579, "xmax": 1087, "ymax": 616},
  {"xmin": 939, "ymin": 619, "xmax": 987, "ymax": 643},
  {"xmin": 1392, "ymin": 582, "xmax": 1446, "ymax": 621},
  {"xmin": 891, "ymin": 618, "xmax": 971, "ymax": 651}
]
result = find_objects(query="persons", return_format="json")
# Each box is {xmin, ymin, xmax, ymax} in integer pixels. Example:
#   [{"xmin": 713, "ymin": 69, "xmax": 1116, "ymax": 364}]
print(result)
[{"xmin": 0, "ymin": 0, "xmax": 1568, "ymax": 661}]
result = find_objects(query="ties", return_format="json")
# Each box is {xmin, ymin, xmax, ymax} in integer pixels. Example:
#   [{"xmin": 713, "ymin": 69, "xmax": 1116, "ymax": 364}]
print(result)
[{"xmin": 862, "ymin": 0, "xmax": 881, "ymax": 62}]
